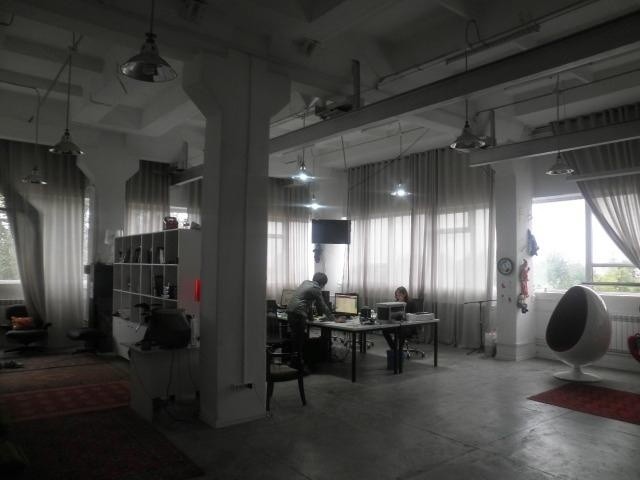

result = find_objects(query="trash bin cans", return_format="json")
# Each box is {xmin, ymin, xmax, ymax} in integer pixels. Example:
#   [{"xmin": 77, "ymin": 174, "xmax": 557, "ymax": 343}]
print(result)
[{"xmin": 386, "ymin": 349, "xmax": 397, "ymax": 371}]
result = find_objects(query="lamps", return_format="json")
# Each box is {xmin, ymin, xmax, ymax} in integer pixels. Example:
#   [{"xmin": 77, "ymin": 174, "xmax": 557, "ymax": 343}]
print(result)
[
  {"xmin": 390, "ymin": 133, "xmax": 414, "ymax": 198},
  {"xmin": 48, "ymin": 47, "xmax": 87, "ymax": 156},
  {"xmin": 121, "ymin": 1, "xmax": 176, "ymax": 81},
  {"xmin": 546, "ymin": 96, "xmax": 574, "ymax": 176},
  {"xmin": 450, "ymin": 96, "xmax": 485, "ymax": 153},
  {"xmin": 293, "ymin": 151, "xmax": 314, "ymax": 182},
  {"xmin": 20, "ymin": 102, "xmax": 48, "ymax": 185}
]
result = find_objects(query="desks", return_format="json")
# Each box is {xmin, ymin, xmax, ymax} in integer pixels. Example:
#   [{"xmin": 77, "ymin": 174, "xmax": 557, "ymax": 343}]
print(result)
[{"xmin": 272, "ymin": 309, "xmax": 440, "ymax": 382}]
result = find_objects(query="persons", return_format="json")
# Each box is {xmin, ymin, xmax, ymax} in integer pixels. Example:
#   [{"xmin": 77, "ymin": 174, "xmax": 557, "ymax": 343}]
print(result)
[
  {"xmin": 285, "ymin": 272, "xmax": 347, "ymax": 377},
  {"xmin": 382, "ymin": 286, "xmax": 416, "ymax": 356}
]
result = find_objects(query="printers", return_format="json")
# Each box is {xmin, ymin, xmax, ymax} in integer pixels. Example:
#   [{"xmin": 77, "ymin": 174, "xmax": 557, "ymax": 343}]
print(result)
[{"xmin": 376, "ymin": 302, "xmax": 408, "ymax": 323}]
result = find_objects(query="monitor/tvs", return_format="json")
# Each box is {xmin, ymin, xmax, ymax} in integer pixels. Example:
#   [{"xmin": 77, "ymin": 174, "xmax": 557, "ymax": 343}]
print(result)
[
  {"xmin": 281, "ymin": 290, "xmax": 295, "ymax": 307},
  {"xmin": 312, "ymin": 219, "xmax": 351, "ymax": 244},
  {"xmin": 335, "ymin": 293, "xmax": 359, "ymax": 319},
  {"xmin": 321, "ymin": 291, "xmax": 330, "ymax": 307}
]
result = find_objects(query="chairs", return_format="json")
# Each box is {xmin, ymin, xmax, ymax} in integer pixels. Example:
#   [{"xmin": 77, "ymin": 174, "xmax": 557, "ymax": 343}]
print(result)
[
  {"xmin": 546, "ymin": 285, "xmax": 612, "ymax": 382},
  {"xmin": 345, "ymin": 297, "xmax": 425, "ymax": 359},
  {"xmin": 266, "ymin": 344, "xmax": 306, "ymax": 408},
  {"xmin": 0, "ymin": 303, "xmax": 52, "ymax": 353}
]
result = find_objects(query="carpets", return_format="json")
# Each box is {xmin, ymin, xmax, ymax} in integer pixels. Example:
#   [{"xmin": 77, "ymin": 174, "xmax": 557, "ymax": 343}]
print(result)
[
  {"xmin": 526, "ymin": 382, "xmax": 640, "ymax": 426},
  {"xmin": 0, "ymin": 353, "xmax": 204, "ymax": 480}
]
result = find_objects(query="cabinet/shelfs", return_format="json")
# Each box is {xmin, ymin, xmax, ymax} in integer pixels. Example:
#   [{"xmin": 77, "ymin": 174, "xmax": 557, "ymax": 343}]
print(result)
[{"xmin": 112, "ymin": 229, "xmax": 201, "ymax": 362}]
